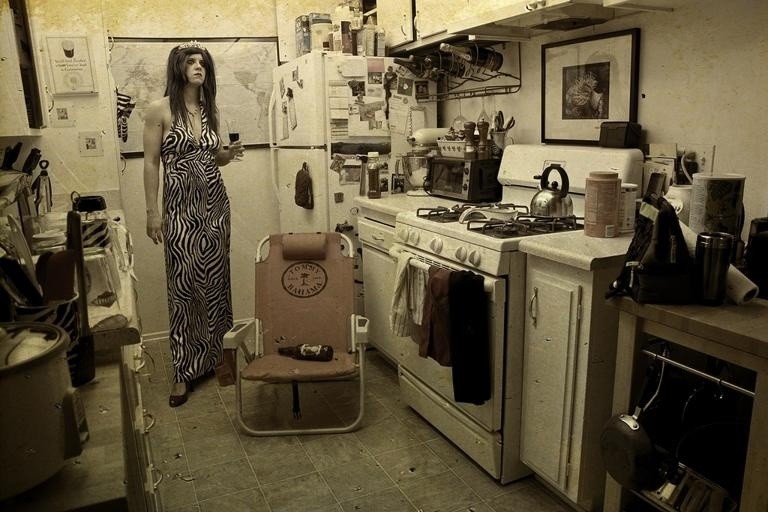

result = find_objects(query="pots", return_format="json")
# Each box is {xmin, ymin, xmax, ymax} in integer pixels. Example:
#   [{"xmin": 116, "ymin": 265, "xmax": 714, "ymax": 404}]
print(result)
[{"xmin": 595, "ymin": 347, "xmax": 665, "ymax": 492}]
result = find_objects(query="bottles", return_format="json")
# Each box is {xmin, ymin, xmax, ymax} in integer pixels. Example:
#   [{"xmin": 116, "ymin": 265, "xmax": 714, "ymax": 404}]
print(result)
[
  {"xmin": 359, "ymin": 150, "xmax": 382, "ymax": 196},
  {"xmin": 331, "ymin": 1, "xmax": 375, "ymax": 56},
  {"xmin": 72, "ymin": 195, "xmax": 127, "ymax": 282},
  {"xmin": 391, "ymin": 43, "xmax": 503, "ymax": 81},
  {"xmin": 461, "ymin": 123, "xmax": 489, "ymax": 158},
  {"xmin": 270, "ymin": 345, "xmax": 336, "ymax": 363},
  {"xmin": 583, "ymin": 172, "xmax": 621, "ymax": 239}
]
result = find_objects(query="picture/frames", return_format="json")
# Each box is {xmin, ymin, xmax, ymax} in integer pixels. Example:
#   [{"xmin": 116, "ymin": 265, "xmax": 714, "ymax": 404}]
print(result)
[{"xmin": 538, "ymin": 28, "xmax": 641, "ymax": 148}]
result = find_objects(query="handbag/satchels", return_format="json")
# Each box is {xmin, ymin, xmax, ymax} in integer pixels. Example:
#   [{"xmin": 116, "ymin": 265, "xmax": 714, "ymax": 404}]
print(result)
[{"xmin": 599, "ymin": 187, "xmax": 704, "ymax": 308}]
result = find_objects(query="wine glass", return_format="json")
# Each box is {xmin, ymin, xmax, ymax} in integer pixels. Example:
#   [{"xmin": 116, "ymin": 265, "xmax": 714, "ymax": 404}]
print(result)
[
  {"xmin": 227, "ymin": 118, "xmax": 240, "ymax": 162},
  {"xmin": 450, "ymin": 94, "xmax": 495, "ymax": 135}
]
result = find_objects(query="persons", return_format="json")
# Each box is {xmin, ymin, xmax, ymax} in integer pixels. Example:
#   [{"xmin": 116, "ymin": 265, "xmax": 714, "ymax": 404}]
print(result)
[{"xmin": 143, "ymin": 42, "xmax": 246, "ymax": 407}]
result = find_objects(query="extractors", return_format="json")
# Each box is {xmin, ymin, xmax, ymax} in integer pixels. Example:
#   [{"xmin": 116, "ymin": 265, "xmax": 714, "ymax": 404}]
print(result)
[{"xmin": 445, "ymin": 0, "xmax": 661, "ymax": 42}]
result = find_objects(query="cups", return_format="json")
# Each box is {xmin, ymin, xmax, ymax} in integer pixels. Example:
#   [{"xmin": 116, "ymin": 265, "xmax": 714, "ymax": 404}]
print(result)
[
  {"xmin": 75, "ymin": 247, "xmax": 126, "ymax": 305},
  {"xmin": 61, "ymin": 41, "xmax": 76, "ymax": 56},
  {"xmin": 490, "ymin": 131, "xmax": 514, "ymax": 157},
  {"xmin": 695, "ymin": 231, "xmax": 734, "ymax": 307}
]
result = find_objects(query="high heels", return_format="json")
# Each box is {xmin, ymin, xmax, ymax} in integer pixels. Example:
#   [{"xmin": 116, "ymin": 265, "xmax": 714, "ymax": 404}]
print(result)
[{"xmin": 169, "ymin": 377, "xmax": 195, "ymax": 407}]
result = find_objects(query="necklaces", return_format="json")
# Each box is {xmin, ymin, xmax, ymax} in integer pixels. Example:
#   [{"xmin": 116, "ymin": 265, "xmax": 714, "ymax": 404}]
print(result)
[{"xmin": 186, "ymin": 104, "xmax": 199, "ymax": 119}]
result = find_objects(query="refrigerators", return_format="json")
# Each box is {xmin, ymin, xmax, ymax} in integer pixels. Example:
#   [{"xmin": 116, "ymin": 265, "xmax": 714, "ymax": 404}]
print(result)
[{"xmin": 271, "ymin": 52, "xmax": 437, "ymax": 352}]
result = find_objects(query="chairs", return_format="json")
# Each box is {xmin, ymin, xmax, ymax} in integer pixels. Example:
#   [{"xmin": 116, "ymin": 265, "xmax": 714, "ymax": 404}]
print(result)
[{"xmin": 220, "ymin": 230, "xmax": 369, "ymax": 436}]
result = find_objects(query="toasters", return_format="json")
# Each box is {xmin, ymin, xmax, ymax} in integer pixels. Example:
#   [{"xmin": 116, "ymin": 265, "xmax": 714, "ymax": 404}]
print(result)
[{"xmin": 422, "ymin": 154, "xmax": 506, "ymax": 202}]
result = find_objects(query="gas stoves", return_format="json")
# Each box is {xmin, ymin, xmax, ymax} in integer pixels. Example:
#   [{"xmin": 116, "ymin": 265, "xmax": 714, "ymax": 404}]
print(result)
[{"xmin": 394, "ymin": 200, "xmax": 586, "ymax": 275}]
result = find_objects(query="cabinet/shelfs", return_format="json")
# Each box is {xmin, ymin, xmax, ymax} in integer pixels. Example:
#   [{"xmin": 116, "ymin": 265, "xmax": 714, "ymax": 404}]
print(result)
[
  {"xmin": 355, "ymin": 216, "xmax": 396, "ymax": 365},
  {"xmin": 518, "ymin": 267, "xmax": 581, "ymax": 497},
  {"xmin": 376, "ymin": 0, "xmax": 444, "ymax": 49},
  {"xmin": 603, "ymin": 293, "xmax": 768, "ymax": 512}
]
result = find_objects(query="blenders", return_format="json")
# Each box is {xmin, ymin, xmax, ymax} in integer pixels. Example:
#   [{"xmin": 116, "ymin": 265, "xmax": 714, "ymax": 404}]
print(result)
[{"xmin": 401, "ymin": 126, "xmax": 452, "ymax": 196}]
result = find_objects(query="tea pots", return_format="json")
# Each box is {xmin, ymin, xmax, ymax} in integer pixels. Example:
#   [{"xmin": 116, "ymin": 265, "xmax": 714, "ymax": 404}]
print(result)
[{"xmin": 529, "ymin": 165, "xmax": 574, "ymax": 218}]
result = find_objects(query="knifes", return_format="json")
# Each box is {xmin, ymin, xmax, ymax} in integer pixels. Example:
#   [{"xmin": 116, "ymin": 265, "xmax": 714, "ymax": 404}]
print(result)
[{"xmin": 4, "ymin": 140, "xmax": 40, "ymax": 174}]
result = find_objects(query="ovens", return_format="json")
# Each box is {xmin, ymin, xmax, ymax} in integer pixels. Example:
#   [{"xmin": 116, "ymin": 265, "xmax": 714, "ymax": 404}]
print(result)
[{"xmin": 390, "ymin": 248, "xmax": 529, "ymax": 482}]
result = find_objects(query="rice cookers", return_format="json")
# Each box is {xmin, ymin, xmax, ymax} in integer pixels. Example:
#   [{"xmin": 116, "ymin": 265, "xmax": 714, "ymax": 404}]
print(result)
[{"xmin": 0, "ymin": 320, "xmax": 91, "ymax": 502}]
